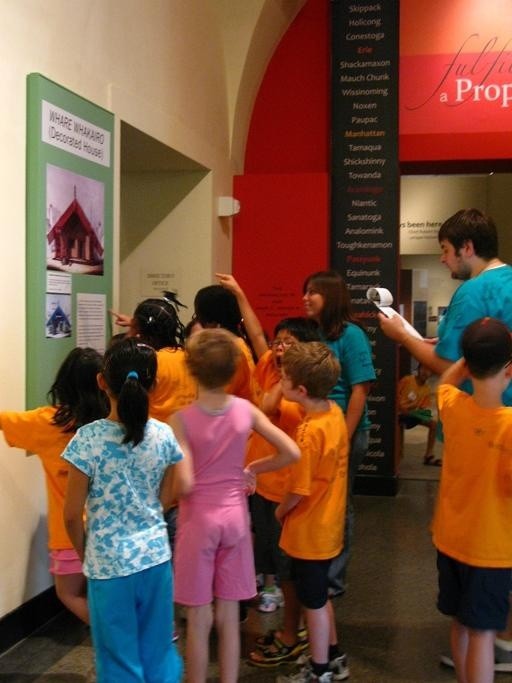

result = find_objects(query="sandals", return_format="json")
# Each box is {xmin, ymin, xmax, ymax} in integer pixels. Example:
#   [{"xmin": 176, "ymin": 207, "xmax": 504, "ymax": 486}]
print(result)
[{"xmin": 423, "ymin": 455, "xmax": 442, "ymax": 467}]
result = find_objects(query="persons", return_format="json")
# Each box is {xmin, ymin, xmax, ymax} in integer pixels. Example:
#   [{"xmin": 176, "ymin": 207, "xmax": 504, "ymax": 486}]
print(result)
[
  {"xmin": 396, "ymin": 362, "xmax": 442, "ymax": 465},
  {"xmin": 59, "ymin": 338, "xmax": 187, "ymax": 683},
  {"xmin": 301, "ymin": 272, "xmax": 374, "ymax": 599},
  {"xmin": 166, "ymin": 330, "xmax": 300, "ymax": 683},
  {"xmin": 366, "ymin": 207, "xmax": 511, "ymax": 438},
  {"xmin": 425, "ymin": 314, "xmax": 510, "ymax": 677},
  {"xmin": 273, "ymin": 340, "xmax": 351, "ymax": 683},
  {"xmin": 1, "ymin": 285, "xmax": 256, "ymax": 630},
  {"xmin": 215, "ymin": 274, "xmax": 324, "ymax": 666}
]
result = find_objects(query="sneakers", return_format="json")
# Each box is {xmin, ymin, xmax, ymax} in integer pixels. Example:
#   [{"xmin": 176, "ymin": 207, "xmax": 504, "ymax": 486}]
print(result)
[
  {"xmin": 441, "ymin": 644, "xmax": 512, "ymax": 673},
  {"xmin": 240, "ymin": 570, "xmax": 349, "ymax": 683}
]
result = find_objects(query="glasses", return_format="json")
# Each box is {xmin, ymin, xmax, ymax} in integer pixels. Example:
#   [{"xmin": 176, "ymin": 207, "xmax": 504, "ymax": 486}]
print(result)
[{"xmin": 269, "ymin": 336, "xmax": 298, "ymax": 349}]
